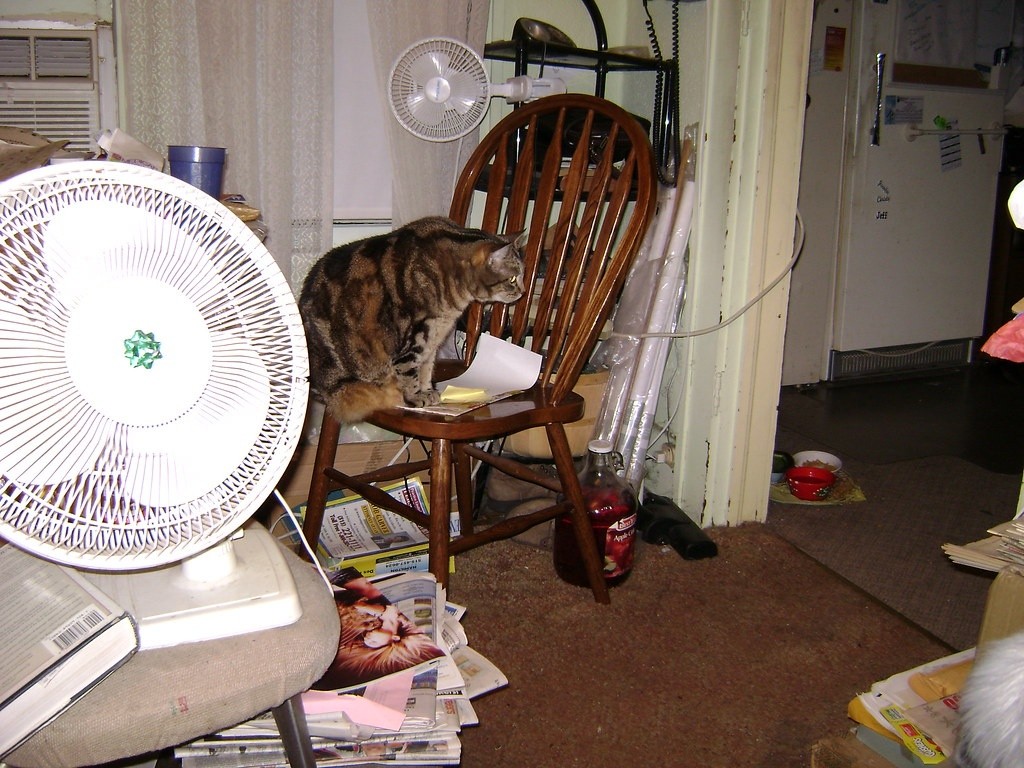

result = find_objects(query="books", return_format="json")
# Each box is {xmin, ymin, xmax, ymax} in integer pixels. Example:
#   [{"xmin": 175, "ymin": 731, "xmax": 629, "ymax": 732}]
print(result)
[
  {"xmin": 942, "ymin": 512, "xmax": 1024, "ymax": 577},
  {"xmin": 0, "ymin": 543, "xmax": 141, "ymax": 759},
  {"xmin": 299, "ymin": 475, "xmax": 455, "ymax": 579},
  {"xmin": 301, "ymin": 566, "xmax": 447, "ymax": 693}
]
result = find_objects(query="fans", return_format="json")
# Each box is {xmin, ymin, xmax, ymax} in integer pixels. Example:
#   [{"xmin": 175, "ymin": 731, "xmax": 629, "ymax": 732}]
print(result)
[
  {"xmin": 0, "ymin": 160, "xmax": 310, "ymax": 652},
  {"xmin": 387, "ymin": 37, "xmax": 571, "ymax": 146}
]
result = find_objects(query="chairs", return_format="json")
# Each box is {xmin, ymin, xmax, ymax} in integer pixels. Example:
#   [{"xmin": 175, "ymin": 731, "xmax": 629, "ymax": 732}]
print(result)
[{"xmin": 298, "ymin": 92, "xmax": 659, "ymax": 609}]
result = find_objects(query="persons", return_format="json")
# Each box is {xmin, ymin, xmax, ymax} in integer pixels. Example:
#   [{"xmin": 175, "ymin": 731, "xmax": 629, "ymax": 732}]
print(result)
[{"xmin": 371, "ymin": 534, "xmax": 410, "ymax": 549}]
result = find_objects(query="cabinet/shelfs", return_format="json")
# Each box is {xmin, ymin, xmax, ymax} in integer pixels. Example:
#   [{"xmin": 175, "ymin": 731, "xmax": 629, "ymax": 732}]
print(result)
[{"xmin": 475, "ymin": 42, "xmax": 679, "ymax": 205}]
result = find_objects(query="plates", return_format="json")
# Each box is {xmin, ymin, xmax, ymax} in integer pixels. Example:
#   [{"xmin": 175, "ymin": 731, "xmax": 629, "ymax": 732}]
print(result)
[{"xmin": 792, "ymin": 450, "xmax": 843, "ymax": 477}]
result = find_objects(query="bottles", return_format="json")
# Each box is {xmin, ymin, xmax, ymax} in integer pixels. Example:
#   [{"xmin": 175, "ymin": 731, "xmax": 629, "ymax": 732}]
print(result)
[{"xmin": 552, "ymin": 439, "xmax": 638, "ymax": 589}]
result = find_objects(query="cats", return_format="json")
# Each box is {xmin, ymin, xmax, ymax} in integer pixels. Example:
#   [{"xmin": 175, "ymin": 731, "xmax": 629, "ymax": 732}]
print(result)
[{"xmin": 298, "ymin": 216, "xmax": 527, "ymax": 425}]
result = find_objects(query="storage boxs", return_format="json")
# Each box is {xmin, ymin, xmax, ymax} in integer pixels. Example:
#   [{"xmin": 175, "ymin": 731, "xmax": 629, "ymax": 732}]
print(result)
[{"xmin": 497, "ymin": 365, "xmax": 614, "ymax": 458}]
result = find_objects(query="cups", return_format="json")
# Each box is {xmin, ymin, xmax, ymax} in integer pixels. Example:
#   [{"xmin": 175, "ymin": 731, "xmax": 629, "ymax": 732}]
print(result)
[{"xmin": 167, "ymin": 145, "xmax": 227, "ymax": 201}]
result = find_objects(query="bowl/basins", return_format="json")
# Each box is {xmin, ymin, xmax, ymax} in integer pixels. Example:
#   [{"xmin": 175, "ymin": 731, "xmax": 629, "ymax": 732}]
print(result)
[
  {"xmin": 770, "ymin": 450, "xmax": 794, "ymax": 484},
  {"xmin": 785, "ymin": 466, "xmax": 836, "ymax": 501},
  {"xmin": 511, "ymin": 16, "xmax": 577, "ymax": 56}
]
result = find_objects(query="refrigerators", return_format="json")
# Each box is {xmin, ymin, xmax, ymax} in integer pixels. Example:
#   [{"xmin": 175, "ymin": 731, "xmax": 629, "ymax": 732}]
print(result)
[{"xmin": 823, "ymin": 0, "xmax": 1017, "ymax": 384}]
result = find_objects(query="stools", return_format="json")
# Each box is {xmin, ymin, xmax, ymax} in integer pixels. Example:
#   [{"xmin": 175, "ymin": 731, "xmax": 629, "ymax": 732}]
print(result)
[{"xmin": 1, "ymin": 535, "xmax": 345, "ymax": 768}]
